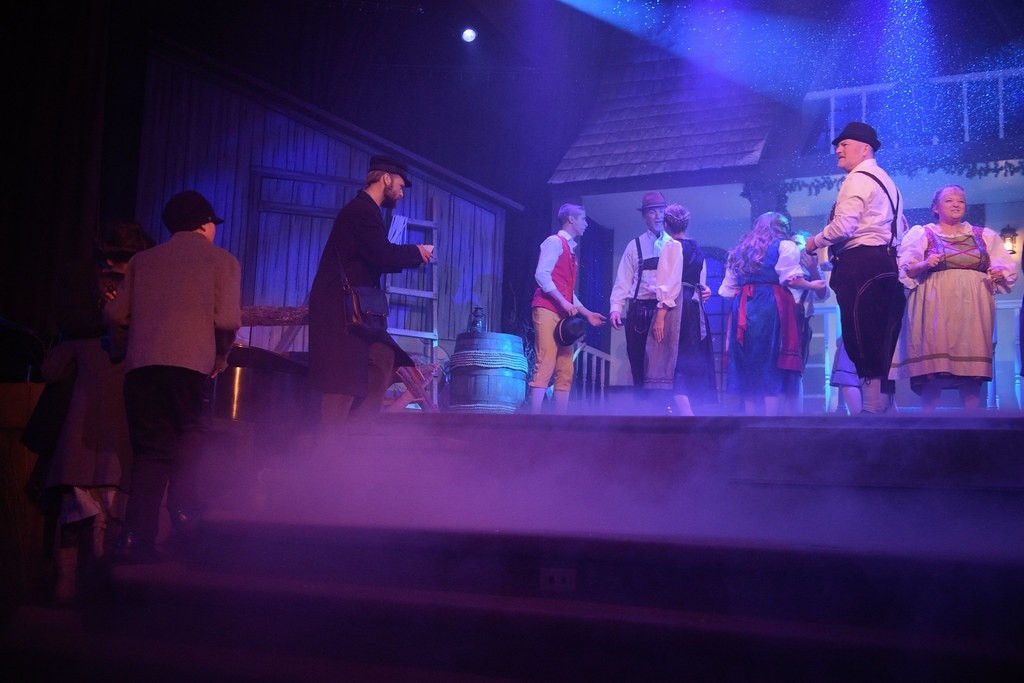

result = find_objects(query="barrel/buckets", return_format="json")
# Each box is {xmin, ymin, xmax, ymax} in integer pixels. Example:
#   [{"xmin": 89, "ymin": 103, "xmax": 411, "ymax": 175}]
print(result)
[{"xmin": 450, "ymin": 331, "xmax": 529, "ymax": 415}]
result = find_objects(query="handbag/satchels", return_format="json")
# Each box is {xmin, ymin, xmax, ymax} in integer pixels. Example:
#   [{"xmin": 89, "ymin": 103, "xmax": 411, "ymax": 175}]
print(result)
[
  {"xmin": 344, "ymin": 284, "xmax": 389, "ymax": 336},
  {"xmin": 20, "ymin": 381, "xmax": 73, "ymax": 454}
]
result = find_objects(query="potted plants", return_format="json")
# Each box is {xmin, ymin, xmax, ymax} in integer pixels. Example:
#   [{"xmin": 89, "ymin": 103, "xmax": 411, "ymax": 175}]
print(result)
[{"xmin": 1, "ymin": 216, "xmax": 107, "ymax": 556}]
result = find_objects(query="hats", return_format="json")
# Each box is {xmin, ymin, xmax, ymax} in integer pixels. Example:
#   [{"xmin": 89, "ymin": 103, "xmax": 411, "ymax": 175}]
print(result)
[
  {"xmin": 161, "ymin": 190, "xmax": 225, "ymax": 232},
  {"xmin": 554, "ymin": 314, "xmax": 587, "ymax": 345},
  {"xmin": 832, "ymin": 122, "xmax": 880, "ymax": 152},
  {"xmin": 368, "ymin": 155, "xmax": 413, "ymax": 189},
  {"xmin": 636, "ymin": 192, "xmax": 668, "ymax": 210},
  {"xmin": 98, "ymin": 223, "xmax": 156, "ymax": 255}
]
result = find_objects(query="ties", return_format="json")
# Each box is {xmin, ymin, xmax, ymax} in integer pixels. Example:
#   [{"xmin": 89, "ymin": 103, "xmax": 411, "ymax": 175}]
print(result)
[{"xmin": 570, "ymin": 253, "xmax": 575, "ymax": 265}]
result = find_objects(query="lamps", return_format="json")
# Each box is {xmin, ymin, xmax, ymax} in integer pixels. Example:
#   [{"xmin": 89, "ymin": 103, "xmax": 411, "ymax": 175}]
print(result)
[{"xmin": 1000, "ymin": 225, "xmax": 1018, "ymax": 254}]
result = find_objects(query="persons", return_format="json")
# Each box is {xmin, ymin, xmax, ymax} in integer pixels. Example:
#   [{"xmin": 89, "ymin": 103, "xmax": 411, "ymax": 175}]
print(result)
[
  {"xmin": 899, "ymin": 184, "xmax": 1019, "ymax": 418},
  {"xmin": 805, "ymin": 121, "xmax": 906, "ymax": 417},
  {"xmin": 104, "ymin": 191, "xmax": 241, "ymax": 565},
  {"xmin": 300, "ymin": 153, "xmax": 437, "ymax": 423},
  {"xmin": 825, "ymin": 321, "xmax": 900, "ymax": 417},
  {"xmin": 517, "ymin": 203, "xmax": 608, "ymax": 417},
  {"xmin": 643, "ymin": 203, "xmax": 718, "ymax": 418},
  {"xmin": 718, "ymin": 211, "xmax": 828, "ymax": 417},
  {"xmin": 610, "ymin": 191, "xmax": 674, "ymax": 408},
  {"xmin": 765, "ymin": 206, "xmax": 831, "ymax": 418}
]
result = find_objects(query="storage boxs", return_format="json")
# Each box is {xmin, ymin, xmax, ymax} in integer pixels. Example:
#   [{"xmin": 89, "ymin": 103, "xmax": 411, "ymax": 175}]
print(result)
[
  {"xmin": 450, "ymin": 332, "xmax": 525, "ymax": 412},
  {"xmin": 215, "ymin": 344, "xmax": 305, "ymax": 421}
]
